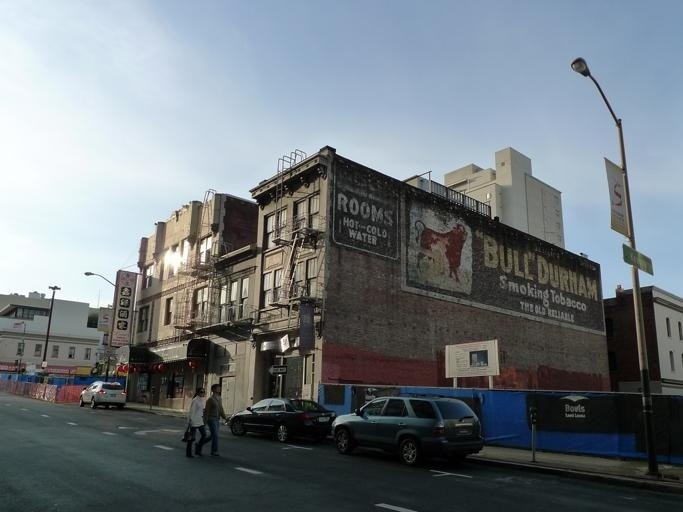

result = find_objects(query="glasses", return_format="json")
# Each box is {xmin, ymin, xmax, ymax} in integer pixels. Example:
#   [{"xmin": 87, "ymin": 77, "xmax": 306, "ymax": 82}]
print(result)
[{"xmin": 199, "ymin": 391, "xmax": 206, "ymax": 394}]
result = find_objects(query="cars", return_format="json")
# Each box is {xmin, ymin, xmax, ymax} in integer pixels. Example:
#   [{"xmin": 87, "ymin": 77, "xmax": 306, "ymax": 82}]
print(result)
[
  {"xmin": 79, "ymin": 381, "xmax": 127, "ymax": 409},
  {"xmin": 229, "ymin": 397, "xmax": 337, "ymax": 442},
  {"xmin": 330, "ymin": 396, "xmax": 485, "ymax": 466}
]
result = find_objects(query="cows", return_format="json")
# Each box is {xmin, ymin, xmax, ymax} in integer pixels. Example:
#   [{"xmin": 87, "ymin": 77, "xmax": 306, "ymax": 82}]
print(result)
[{"xmin": 415, "ymin": 220, "xmax": 468, "ymax": 283}]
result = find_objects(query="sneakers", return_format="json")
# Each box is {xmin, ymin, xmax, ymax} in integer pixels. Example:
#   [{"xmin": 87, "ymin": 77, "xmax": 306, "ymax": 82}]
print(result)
[
  {"xmin": 195, "ymin": 442, "xmax": 199, "ymax": 446},
  {"xmin": 209, "ymin": 452, "xmax": 220, "ymax": 456}
]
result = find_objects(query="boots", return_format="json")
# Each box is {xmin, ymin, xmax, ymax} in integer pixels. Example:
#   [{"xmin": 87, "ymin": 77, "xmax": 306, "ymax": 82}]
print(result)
[
  {"xmin": 195, "ymin": 447, "xmax": 204, "ymax": 457},
  {"xmin": 186, "ymin": 450, "xmax": 194, "ymax": 458}
]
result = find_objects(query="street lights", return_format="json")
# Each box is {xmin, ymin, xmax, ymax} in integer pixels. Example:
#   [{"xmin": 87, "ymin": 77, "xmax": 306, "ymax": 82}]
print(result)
[
  {"xmin": 84, "ymin": 271, "xmax": 120, "ymax": 383},
  {"xmin": 569, "ymin": 58, "xmax": 661, "ymax": 477},
  {"xmin": 11, "ymin": 321, "xmax": 26, "ymax": 379}
]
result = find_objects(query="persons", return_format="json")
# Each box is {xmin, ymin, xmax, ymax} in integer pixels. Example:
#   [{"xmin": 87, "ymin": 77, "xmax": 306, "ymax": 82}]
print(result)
[{"xmin": 186, "ymin": 384, "xmax": 230, "ymax": 458}]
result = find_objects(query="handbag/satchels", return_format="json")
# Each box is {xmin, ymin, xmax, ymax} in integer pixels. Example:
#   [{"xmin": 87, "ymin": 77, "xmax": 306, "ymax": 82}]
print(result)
[{"xmin": 182, "ymin": 432, "xmax": 195, "ymax": 443}]
